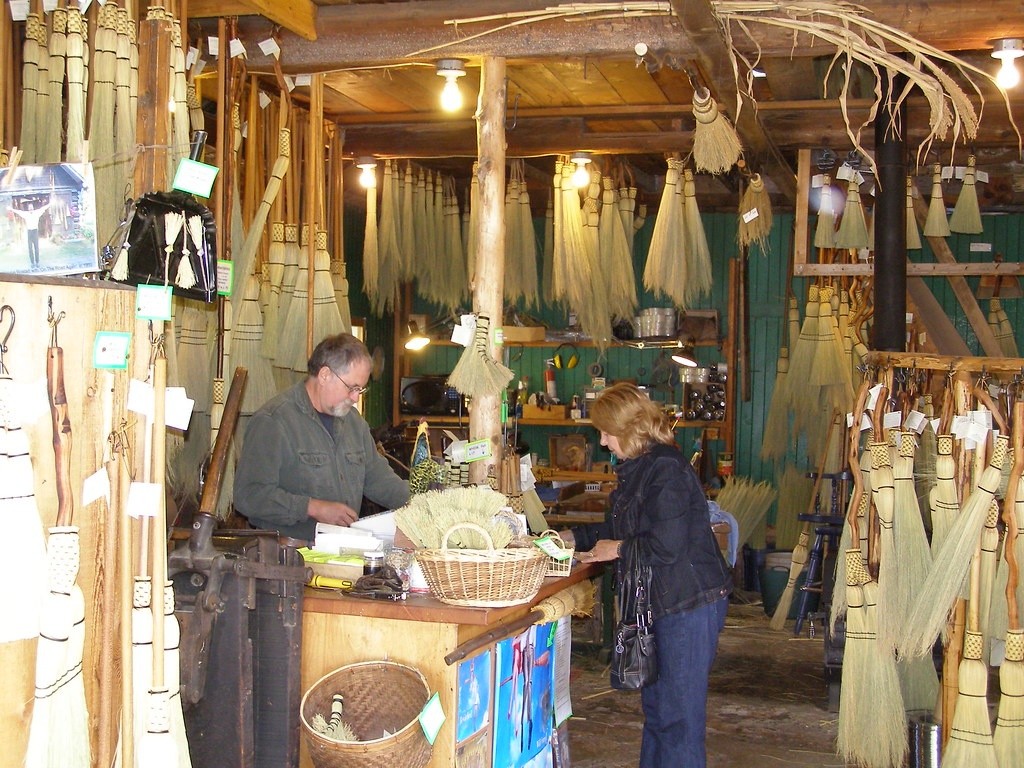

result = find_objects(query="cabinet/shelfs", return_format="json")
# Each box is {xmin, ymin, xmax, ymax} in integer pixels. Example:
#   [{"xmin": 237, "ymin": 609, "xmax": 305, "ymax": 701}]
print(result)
[{"xmin": 392, "ymin": 256, "xmax": 742, "ymax": 605}]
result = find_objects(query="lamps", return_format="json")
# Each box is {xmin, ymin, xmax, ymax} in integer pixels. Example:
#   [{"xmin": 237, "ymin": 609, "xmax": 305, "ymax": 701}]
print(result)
[
  {"xmin": 403, "ymin": 316, "xmax": 431, "ymax": 350},
  {"xmin": 671, "ymin": 336, "xmax": 703, "ymax": 370}
]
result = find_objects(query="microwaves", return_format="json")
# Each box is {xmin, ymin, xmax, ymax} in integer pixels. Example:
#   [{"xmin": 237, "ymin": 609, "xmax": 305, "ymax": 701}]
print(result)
[{"xmin": 400, "ymin": 376, "xmax": 466, "ymax": 417}]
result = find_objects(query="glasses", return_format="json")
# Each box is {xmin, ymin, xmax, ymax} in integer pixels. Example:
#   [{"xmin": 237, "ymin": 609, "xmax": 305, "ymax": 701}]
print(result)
[{"xmin": 330, "ymin": 368, "xmax": 368, "ymax": 394}]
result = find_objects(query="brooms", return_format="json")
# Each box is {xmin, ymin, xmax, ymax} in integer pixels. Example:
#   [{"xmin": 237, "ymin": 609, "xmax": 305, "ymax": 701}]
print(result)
[{"xmin": 0, "ymin": 1, "xmax": 1024, "ymax": 768}]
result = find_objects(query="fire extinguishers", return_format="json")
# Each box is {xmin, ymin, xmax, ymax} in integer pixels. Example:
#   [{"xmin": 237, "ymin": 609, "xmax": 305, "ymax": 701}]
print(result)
[{"xmin": 543, "ymin": 359, "xmax": 559, "ymax": 405}]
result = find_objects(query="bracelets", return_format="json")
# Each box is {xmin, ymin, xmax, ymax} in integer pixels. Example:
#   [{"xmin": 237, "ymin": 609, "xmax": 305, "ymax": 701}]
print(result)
[{"xmin": 617, "ymin": 542, "xmax": 622, "ymax": 558}]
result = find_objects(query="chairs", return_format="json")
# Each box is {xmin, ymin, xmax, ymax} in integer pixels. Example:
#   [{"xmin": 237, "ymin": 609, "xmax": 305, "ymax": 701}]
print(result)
[{"xmin": 795, "ymin": 468, "xmax": 854, "ymax": 637}]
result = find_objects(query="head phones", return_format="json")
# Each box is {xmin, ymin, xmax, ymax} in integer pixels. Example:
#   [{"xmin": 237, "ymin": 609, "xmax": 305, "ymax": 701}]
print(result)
[{"xmin": 553, "ymin": 341, "xmax": 580, "ymax": 369}]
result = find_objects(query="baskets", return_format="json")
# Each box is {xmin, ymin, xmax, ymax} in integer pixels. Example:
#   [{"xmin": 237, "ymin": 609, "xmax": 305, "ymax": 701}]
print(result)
[
  {"xmin": 414, "ymin": 523, "xmax": 551, "ymax": 608},
  {"xmin": 584, "ymin": 481, "xmax": 600, "ymax": 491},
  {"xmin": 540, "ymin": 530, "xmax": 574, "ymax": 577},
  {"xmin": 300, "ymin": 661, "xmax": 433, "ymax": 768}
]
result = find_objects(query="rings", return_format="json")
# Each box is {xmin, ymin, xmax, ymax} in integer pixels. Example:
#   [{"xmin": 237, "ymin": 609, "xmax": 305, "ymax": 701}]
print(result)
[{"xmin": 590, "ymin": 553, "xmax": 593, "ymax": 556}]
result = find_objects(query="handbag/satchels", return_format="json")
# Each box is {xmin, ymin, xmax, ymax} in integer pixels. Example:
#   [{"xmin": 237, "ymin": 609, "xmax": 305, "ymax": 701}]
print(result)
[{"xmin": 610, "ymin": 619, "xmax": 658, "ymax": 691}]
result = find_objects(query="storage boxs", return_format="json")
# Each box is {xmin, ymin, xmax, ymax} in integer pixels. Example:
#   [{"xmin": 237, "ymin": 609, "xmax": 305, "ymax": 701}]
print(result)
[
  {"xmin": 503, "ymin": 325, "xmax": 545, "ymax": 342},
  {"xmin": 522, "ymin": 404, "xmax": 569, "ymax": 421}
]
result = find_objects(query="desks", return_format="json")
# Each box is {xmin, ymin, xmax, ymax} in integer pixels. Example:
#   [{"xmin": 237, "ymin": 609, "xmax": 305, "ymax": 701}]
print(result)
[{"xmin": 302, "ymin": 557, "xmax": 609, "ymax": 768}]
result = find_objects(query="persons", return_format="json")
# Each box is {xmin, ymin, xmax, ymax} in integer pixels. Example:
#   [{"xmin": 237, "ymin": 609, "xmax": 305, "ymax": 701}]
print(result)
[
  {"xmin": 550, "ymin": 380, "xmax": 736, "ymax": 768},
  {"xmin": 507, "ymin": 623, "xmax": 536, "ymax": 754},
  {"xmin": 6, "ymin": 202, "xmax": 51, "ymax": 268},
  {"xmin": 233, "ymin": 332, "xmax": 411, "ymax": 541}
]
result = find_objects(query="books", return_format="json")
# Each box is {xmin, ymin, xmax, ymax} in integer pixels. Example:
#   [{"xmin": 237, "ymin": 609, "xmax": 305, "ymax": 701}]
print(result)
[{"xmin": 552, "ymin": 720, "xmax": 570, "ymax": 768}]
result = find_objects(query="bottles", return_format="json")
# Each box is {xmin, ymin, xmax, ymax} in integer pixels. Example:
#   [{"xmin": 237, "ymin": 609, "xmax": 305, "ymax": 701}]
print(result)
[
  {"xmin": 685, "ymin": 364, "xmax": 727, "ymax": 423},
  {"xmin": 363, "ymin": 552, "xmax": 384, "ymax": 576},
  {"xmin": 545, "ymin": 369, "xmax": 557, "ymax": 398},
  {"xmin": 517, "ymin": 375, "xmax": 529, "ymax": 406}
]
